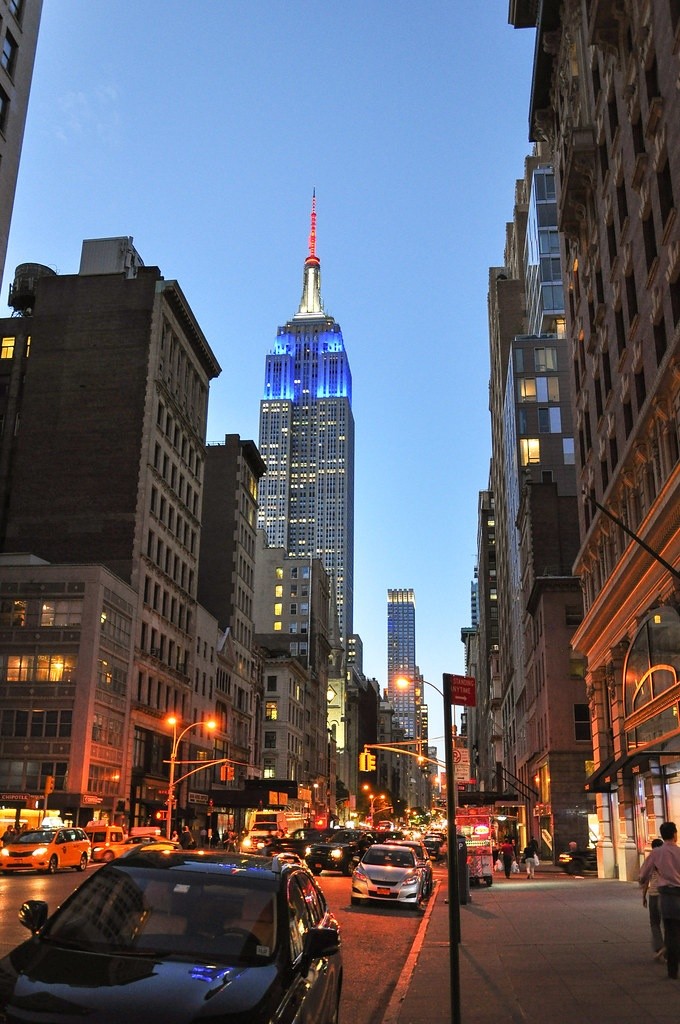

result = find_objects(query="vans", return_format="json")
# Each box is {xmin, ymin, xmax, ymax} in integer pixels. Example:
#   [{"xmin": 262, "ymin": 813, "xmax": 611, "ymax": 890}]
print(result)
[{"xmin": 83, "ymin": 826, "xmax": 125, "ymax": 860}]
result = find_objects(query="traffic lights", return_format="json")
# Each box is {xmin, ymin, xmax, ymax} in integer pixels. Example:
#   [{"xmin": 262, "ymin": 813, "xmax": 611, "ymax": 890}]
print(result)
[
  {"xmin": 366, "ymin": 753, "xmax": 376, "ymax": 771},
  {"xmin": 156, "ymin": 810, "xmax": 162, "ymax": 819}
]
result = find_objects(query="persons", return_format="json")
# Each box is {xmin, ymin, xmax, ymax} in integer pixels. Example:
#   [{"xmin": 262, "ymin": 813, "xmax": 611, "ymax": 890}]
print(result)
[
  {"xmin": 20, "ymin": 824, "xmax": 28, "ymax": 834},
  {"xmin": 510, "ymin": 839, "xmax": 520, "ymax": 864},
  {"xmin": 221, "ymin": 829, "xmax": 243, "ymax": 854},
  {"xmin": 171, "ymin": 831, "xmax": 179, "ymax": 841},
  {"xmin": 500, "ymin": 839, "xmax": 515, "ymax": 879},
  {"xmin": 180, "ymin": 826, "xmax": 195, "ymax": 850},
  {"xmin": 530, "ymin": 836, "xmax": 539, "ymax": 856},
  {"xmin": 200, "ymin": 827, "xmax": 220, "ymax": 848},
  {"xmin": 637, "ymin": 821, "xmax": 680, "ymax": 979},
  {"xmin": 261, "ymin": 826, "xmax": 289, "ymax": 857},
  {"xmin": 0, "ymin": 825, "xmax": 16, "ymax": 848},
  {"xmin": 524, "ymin": 842, "xmax": 537, "ymax": 879},
  {"xmin": 639, "ymin": 838, "xmax": 667, "ymax": 962}
]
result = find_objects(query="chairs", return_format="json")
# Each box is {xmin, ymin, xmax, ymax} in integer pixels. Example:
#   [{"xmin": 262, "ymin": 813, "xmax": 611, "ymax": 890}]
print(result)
[
  {"xmin": 117, "ymin": 880, "xmax": 188, "ymax": 952},
  {"xmin": 224, "ymin": 890, "xmax": 274, "ymax": 950}
]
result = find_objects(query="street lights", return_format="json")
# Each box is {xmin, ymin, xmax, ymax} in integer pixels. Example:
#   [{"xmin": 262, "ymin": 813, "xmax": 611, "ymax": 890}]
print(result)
[
  {"xmin": 397, "ymin": 670, "xmax": 462, "ymax": 1024},
  {"xmin": 165, "ymin": 716, "xmax": 217, "ymax": 840}
]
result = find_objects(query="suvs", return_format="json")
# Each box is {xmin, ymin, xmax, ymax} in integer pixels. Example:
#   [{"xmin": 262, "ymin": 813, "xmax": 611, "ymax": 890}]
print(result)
[{"xmin": 0, "ymin": 841, "xmax": 345, "ymax": 1024}]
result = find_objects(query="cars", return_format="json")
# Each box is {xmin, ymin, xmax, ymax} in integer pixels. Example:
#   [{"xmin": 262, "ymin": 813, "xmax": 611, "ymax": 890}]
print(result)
[
  {"xmin": 0, "ymin": 826, "xmax": 93, "ymax": 875},
  {"xmin": 238, "ymin": 818, "xmax": 447, "ymax": 913},
  {"xmin": 93, "ymin": 833, "xmax": 184, "ymax": 865},
  {"xmin": 556, "ymin": 847, "xmax": 597, "ymax": 876}
]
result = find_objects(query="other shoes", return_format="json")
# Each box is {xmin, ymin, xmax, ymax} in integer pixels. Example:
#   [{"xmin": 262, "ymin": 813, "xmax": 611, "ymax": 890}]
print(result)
[
  {"xmin": 527, "ymin": 873, "xmax": 531, "ymax": 879},
  {"xmin": 654, "ymin": 945, "xmax": 668, "ymax": 960}
]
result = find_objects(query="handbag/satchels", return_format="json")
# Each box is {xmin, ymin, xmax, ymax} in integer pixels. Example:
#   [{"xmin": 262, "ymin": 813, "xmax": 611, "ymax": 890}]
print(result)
[
  {"xmin": 534, "ymin": 853, "xmax": 540, "ymax": 867},
  {"xmin": 511, "ymin": 860, "xmax": 520, "ymax": 874},
  {"xmin": 494, "ymin": 860, "xmax": 504, "ymax": 871}
]
